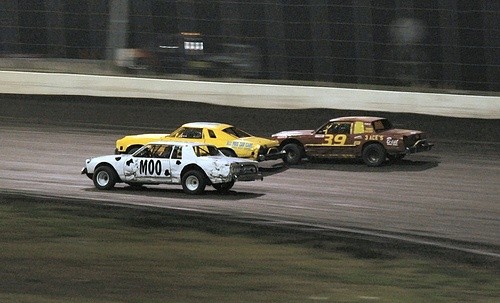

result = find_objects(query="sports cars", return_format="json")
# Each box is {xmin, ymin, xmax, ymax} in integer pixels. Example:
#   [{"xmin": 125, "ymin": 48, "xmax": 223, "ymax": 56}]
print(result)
[
  {"xmin": 79, "ymin": 141, "xmax": 264, "ymax": 194},
  {"xmin": 271, "ymin": 116, "xmax": 435, "ymax": 167},
  {"xmin": 115, "ymin": 121, "xmax": 287, "ymax": 162}
]
